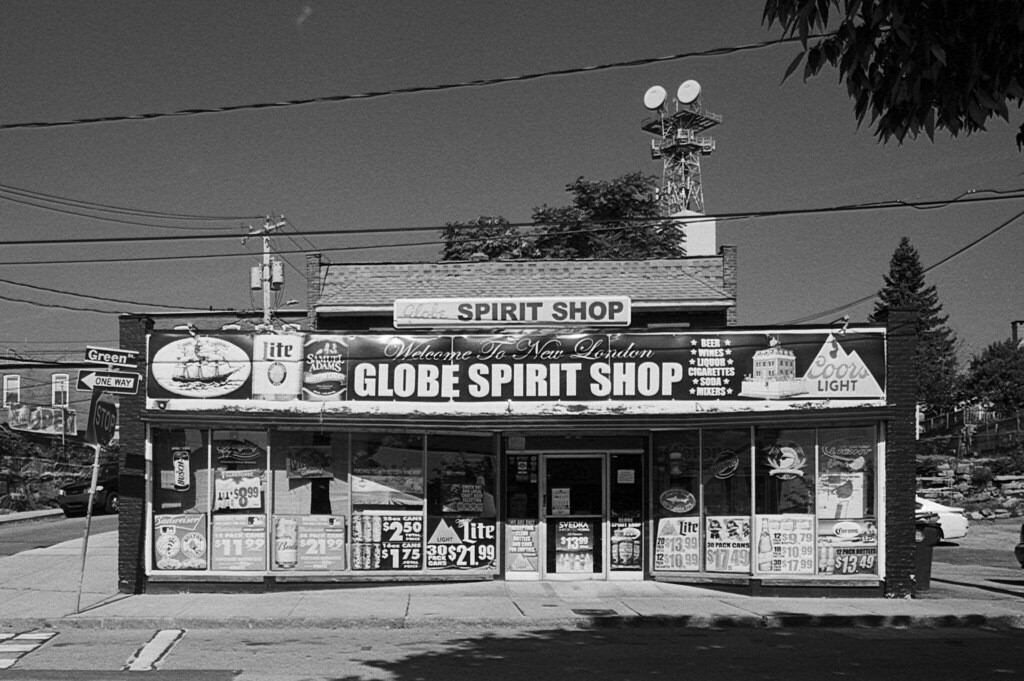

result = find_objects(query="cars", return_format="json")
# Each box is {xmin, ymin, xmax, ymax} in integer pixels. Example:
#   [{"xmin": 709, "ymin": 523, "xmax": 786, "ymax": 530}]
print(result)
[
  {"xmin": 57, "ymin": 461, "xmax": 120, "ymax": 518},
  {"xmin": 915, "ymin": 495, "xmax": 969, "ymax": 543}
]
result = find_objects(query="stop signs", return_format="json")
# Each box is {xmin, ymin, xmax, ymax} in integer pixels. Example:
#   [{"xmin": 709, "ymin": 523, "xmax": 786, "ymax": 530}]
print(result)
[{"xmin": 88, "ymin": 393, "xmax": 118, "ymax": 447}]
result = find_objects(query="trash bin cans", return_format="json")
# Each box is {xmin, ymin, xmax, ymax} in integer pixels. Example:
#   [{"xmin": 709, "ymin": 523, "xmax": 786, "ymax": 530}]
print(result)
[{"xmin": 914, "ymin": 512, "xmax": 941, "ymax": 590}]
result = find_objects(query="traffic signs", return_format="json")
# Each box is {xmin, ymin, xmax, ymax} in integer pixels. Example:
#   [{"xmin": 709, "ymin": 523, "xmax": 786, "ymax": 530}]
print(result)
[{"xmin": 76, "ymin": 345, "xmax": 140, "ymax": 396}]
